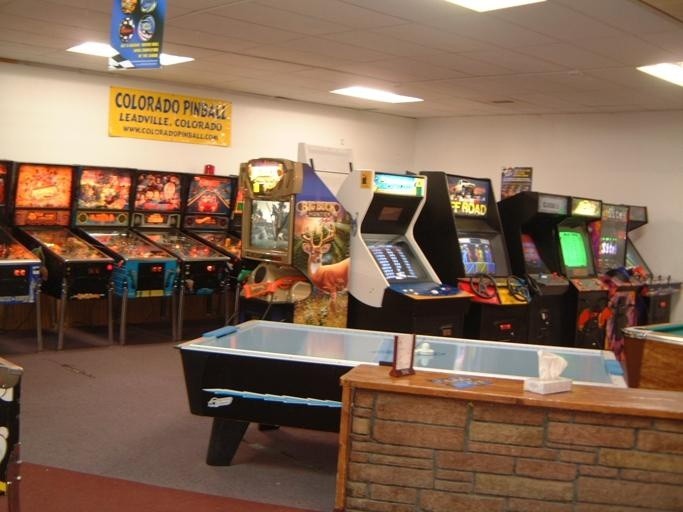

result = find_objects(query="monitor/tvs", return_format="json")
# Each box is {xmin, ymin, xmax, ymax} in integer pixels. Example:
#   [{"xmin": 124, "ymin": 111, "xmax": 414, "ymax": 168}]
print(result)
[
  {"xmin": 521, "ymin": 232, "xmax": 544, "ymax": 270},
  {"xmin": 363, "ymin": 238, "xmax": 423, "ymax": 284},
  {"xmin": 246, "ymin": 194, "xmax": 292, "ymax": 256},
  {"xmin": 458, "ymin": 234, "xmax": 497, "ymax": 276},
  {"xmin": 559, "ymin": 229, "xmax": 589, "ymax": 269}
]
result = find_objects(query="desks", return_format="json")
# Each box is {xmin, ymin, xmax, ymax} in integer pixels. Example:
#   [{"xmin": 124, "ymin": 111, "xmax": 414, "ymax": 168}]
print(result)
[
  {"xmin": 619, "ymin": 322, "xmax": 683, "ymax": 391},
  {"xmin": 172, "ymin": 318, "xmax": 630, "ymax": 466}
]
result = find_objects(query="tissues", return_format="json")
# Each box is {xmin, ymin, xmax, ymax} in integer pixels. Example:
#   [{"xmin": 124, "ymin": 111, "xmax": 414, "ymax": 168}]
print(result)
[{"xmin": 526, "ymin": 351, "xmax": 572, "ymax": 394}]
